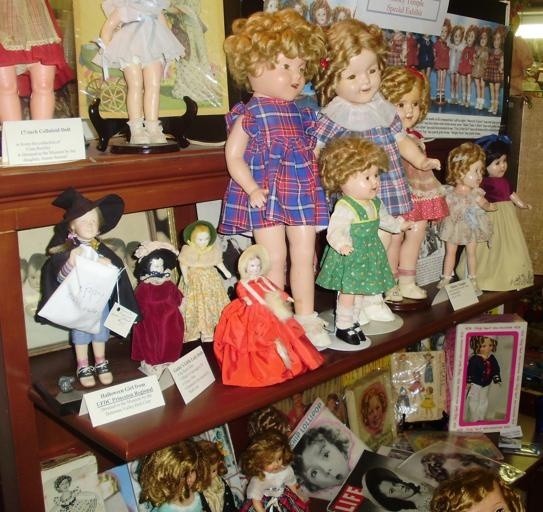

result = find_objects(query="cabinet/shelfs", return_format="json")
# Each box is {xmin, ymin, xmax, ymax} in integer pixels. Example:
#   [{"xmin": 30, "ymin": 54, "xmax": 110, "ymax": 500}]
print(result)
[{"xmin": 0, "ymin": 140, "xmax": 543, "ymax": 512}]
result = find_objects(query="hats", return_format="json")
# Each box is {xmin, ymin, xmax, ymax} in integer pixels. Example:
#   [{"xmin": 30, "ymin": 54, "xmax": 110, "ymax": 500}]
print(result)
[{"xmin": 361, "ymin": 467, "xmax": 402, "ymax": 512}]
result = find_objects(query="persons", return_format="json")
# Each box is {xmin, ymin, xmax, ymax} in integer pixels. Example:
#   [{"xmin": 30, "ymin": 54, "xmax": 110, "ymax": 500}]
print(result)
[
  {"xmin": 0, "ymin": 0, "xmax": 543, "ymax": 512},
  {"xmin": 0, "ymin": 1, "xmax": 533, "ymax": 511}
]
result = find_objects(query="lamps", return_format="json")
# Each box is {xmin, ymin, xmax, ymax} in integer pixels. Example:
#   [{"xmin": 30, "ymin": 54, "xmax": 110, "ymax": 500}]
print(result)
[{"xmin": 511, "ymin": 9, "xmax": 543, "ymax": 41}]
